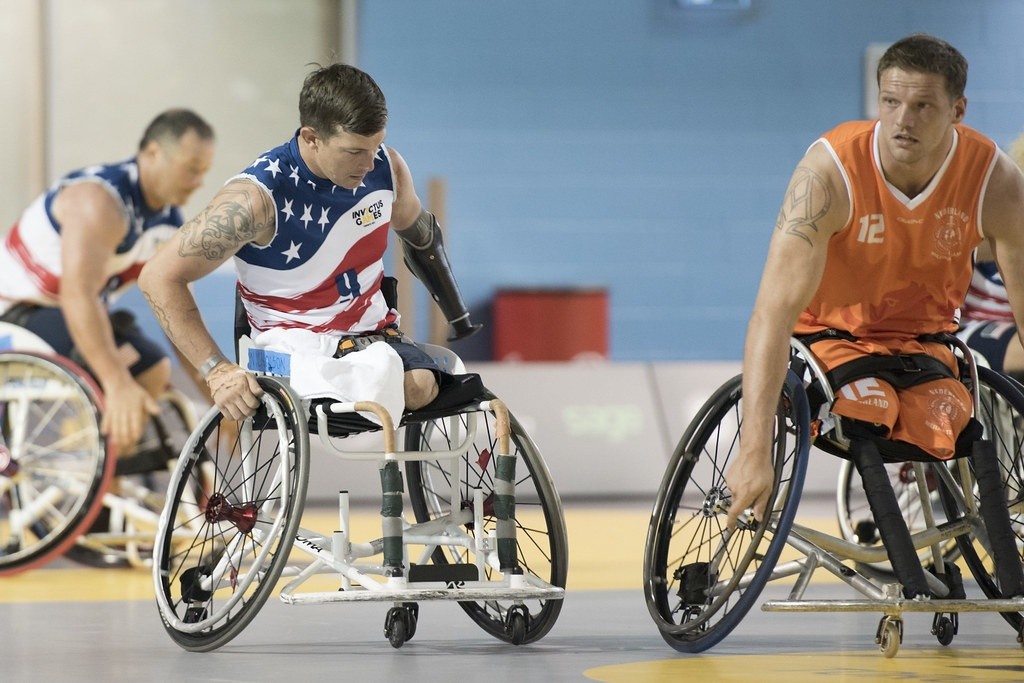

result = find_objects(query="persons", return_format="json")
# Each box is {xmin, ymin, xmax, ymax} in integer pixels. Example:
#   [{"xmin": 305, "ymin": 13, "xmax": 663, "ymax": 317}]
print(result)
[
  {"xmin": 136, "ymin": 63, "xmax": 483, "ymax": 429},
  {"xmin": 0, "ymin": 109, "xmax": 228, "ymax": 458},
  {"xmin": 724, "ymin": 33, "xmax": 1024, "ymax": 532}
]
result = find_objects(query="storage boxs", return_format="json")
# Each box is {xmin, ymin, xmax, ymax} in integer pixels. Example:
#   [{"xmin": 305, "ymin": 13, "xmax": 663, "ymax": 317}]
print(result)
[{"xmin": 494, "ymin": 289, "xmax": 611, "ymax": 359}]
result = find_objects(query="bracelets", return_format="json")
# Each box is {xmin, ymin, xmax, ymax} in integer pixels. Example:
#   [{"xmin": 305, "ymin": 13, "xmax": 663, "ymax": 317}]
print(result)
[{"xmin": 192, "ymin": 353, "xmax": 226, "ymax": 376}]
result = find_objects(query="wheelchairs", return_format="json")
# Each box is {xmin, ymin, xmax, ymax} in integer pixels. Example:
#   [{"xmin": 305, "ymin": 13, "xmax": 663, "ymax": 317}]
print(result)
[
  {"xmin": 0, "ymin": 322, "xmax": 237, "ymax": 578},
  {"xmin": 152, "ymin": 276, "xmax": 571, "ymax": 653},
  {"xmin": 644, "ymin": 327, "xmax": 1023, "ymax": 655}
]
